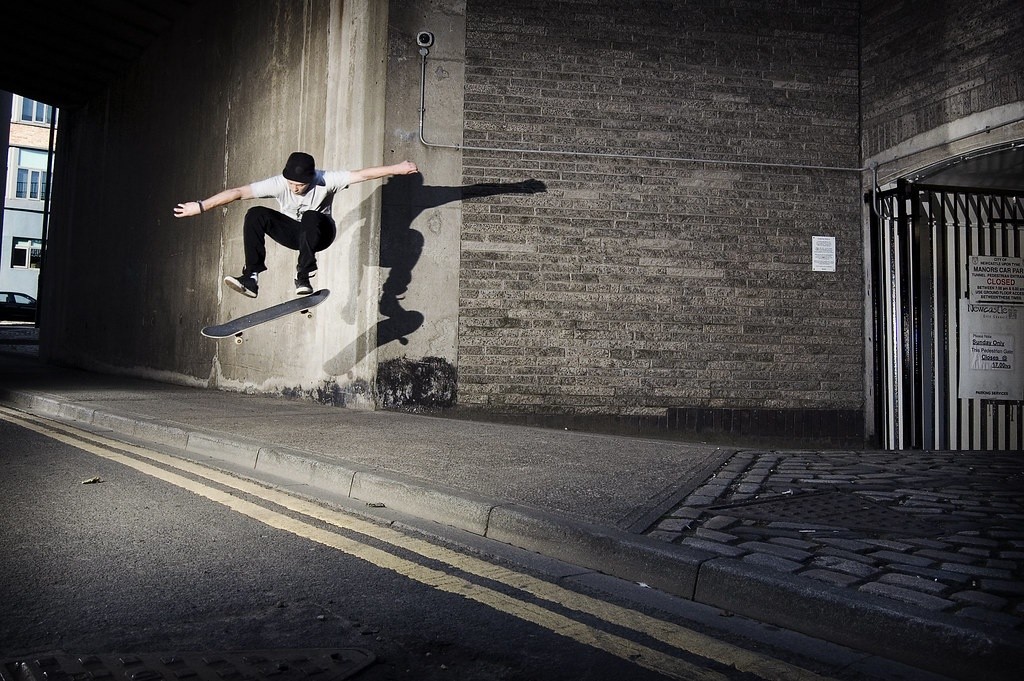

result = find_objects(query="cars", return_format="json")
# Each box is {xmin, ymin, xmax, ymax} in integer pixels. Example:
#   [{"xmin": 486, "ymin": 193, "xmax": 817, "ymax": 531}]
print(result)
[{"xmin": 0, "ymin": 290, "xmax": 37, "ymax": 327}]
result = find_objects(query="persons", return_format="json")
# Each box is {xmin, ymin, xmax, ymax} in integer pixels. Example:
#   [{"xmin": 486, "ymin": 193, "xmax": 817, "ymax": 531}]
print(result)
[{"xmin": 173, "ymin": 152, "xmax": 419, "ymax": 298}]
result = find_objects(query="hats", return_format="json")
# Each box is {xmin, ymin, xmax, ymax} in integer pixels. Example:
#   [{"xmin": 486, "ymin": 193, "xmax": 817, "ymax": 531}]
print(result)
[{"xmin": 283, "ymin": 152, "xmax": 315, "ymax": 182}]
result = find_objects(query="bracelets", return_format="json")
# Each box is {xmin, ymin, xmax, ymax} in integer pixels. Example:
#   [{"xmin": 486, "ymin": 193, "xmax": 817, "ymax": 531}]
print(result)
[{"xmin": 197, "ymin": 200, "xmax": 204, "ymax": 213}]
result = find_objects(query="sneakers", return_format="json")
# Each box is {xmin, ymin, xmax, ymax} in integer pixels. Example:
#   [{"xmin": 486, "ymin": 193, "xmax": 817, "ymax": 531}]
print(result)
[
  {"xmin": 224, "ymin": 267, "xmax": 258, "ymax": 298},
  {"xmin": 294, "ymin": 272, "xmax": 315, "ymax": 294}
]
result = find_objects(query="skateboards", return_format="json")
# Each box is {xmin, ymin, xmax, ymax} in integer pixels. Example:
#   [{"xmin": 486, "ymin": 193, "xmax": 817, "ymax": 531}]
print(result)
[{"xmin": 201, "ymin": 288, "xmax": 331, "ymax": 345}]
